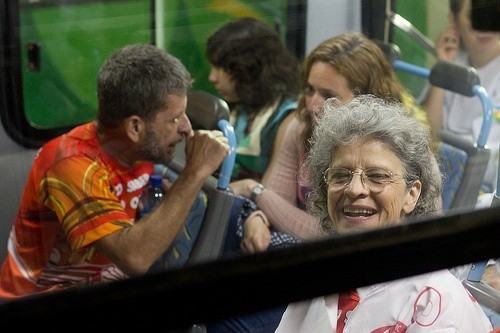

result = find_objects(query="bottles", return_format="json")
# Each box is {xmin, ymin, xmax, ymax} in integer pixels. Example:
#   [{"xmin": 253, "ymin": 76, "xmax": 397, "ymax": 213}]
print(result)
[{"xmin": 138, "ymin": 175, "xmax": 165, "ymax": 222}]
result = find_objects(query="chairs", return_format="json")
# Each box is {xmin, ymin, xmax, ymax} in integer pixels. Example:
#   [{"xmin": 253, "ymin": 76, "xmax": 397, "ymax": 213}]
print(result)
[{"xmin": 141, "ymin": 89, "xmax": 237, "ymax": 332}]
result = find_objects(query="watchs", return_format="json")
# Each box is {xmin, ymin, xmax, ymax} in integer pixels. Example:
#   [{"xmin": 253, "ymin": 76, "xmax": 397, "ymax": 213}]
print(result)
[{"xmin": 250, "ymin": 185, "xmax": 265, "ymax": 200}]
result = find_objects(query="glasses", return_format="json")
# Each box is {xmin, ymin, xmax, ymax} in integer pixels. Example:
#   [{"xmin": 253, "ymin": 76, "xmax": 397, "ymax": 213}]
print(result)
[{"xmin": 321, "ymin": 166, "xmax": 408, "ymax": 187}]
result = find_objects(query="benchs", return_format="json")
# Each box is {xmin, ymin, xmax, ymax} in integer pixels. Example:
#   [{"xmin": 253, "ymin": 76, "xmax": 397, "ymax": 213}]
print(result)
[{"xmin": 374, "ymin": 39, "xmax": 493, "ymax": 210}]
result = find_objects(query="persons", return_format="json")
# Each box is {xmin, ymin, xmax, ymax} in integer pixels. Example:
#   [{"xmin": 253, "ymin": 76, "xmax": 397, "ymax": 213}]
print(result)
[
  {"xmin": 272, "ymin": 93, "xmax": 494, "ymax": 333},
  {"xmin": 0, "ymin": 41, "xmax": 233, "ymax": 309},
  {"xmin": 415, "ymin": 0, "xmax": 500, "ymax": 195},
  {"xmin": 209, "ymin": 31, "xmax": 448, "ymax": 333},
  {"xmin": 443, "ymin": 189, "xmax": 500, "ymax": 294},
  {"xmin": 202, "ymin": 17, "xmax": 310, "ymax": 251}
]
